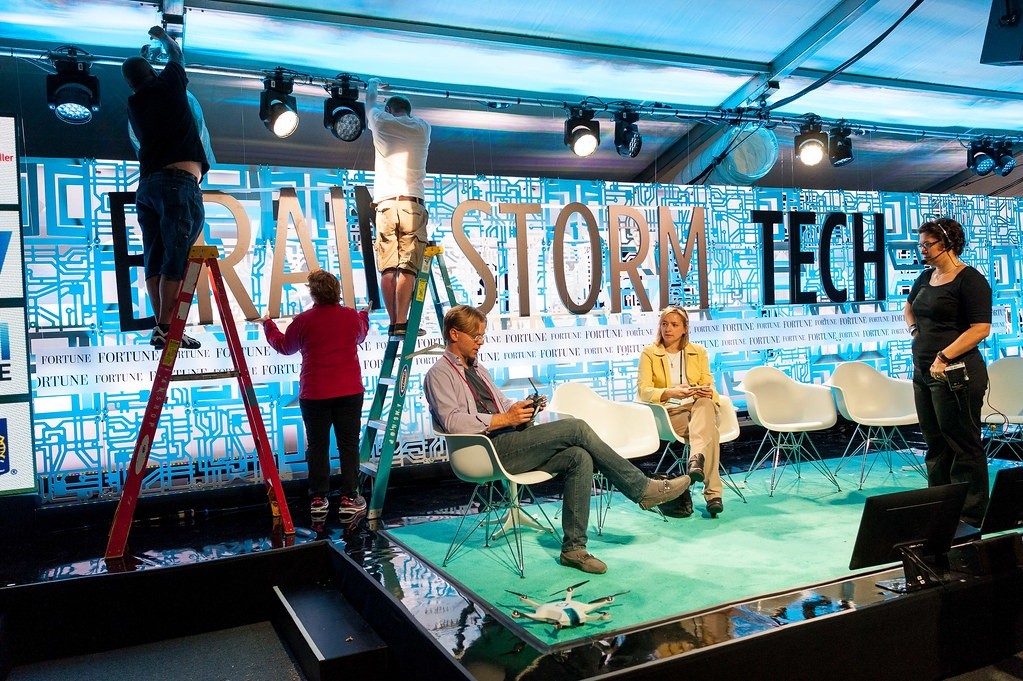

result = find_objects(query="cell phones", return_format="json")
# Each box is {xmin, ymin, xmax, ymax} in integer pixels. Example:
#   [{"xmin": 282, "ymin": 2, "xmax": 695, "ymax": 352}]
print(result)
[{"xmin": 688, "ymin": 386, "xmax": 710, "ymax": 391}]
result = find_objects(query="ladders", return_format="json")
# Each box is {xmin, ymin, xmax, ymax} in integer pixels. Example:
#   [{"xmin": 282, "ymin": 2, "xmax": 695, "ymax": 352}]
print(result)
[
  {"xmin": 359, "ymin": 241, "xmax": 515, "ymax": 530},
  {"xmin": 105, "ymin": 246, "xmax": 295, "ymax": 558}
]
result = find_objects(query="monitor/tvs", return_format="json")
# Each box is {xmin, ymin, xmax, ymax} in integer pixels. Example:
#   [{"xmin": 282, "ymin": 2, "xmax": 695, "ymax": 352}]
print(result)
[
  {"xmin": 849, "ymin": 480, "xmax": 968, "ymax": 593},
  {"xmin": 980, "ymin": 465, "xmax": 1023, "ymax": 534}
]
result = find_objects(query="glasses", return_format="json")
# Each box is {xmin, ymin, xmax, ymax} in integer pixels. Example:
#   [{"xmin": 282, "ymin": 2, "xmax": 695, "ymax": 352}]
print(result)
[
  {"xmin": 916, "ymin": 238, "xmax": 943, "ymax": 251},
  {"xmin": 449, "ymin": 327, "xmax": 486, "ymax": 342}
]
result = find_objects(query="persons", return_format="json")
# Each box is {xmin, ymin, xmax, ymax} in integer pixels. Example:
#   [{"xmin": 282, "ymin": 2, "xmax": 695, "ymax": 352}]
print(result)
[
  {"xmin": 905, "ymin": 219, "xmax": 993, "ymax": 527},
  {"xmin": 122, "ymin": 27, "xmax": 210, "ymax": 349},
  {"xmin": 366, "ymin": 78, "xmax": 432, "ymax": 337},
  {"xmin": 244, "ymin": 269, "xmax": 374, "ymax": 524},
  {"xmin": 423, "ymin": 305, "xmax": 691, "ymax": 574},
  {"xmin": 637, "ymin": 307, "xmax": 723, "ymax": 519}
]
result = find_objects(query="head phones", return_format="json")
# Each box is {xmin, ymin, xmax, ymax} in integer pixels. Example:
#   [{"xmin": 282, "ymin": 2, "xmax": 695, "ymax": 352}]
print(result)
[{"xmin": 932, "ymin": 221, "xmax": 954, "ymax": 250}]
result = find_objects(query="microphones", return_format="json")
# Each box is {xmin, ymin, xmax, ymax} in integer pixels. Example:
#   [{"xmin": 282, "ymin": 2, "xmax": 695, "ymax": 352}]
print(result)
[{"xmin": 929, "ymin": 246, "xmax": 951, "ymax": 262}]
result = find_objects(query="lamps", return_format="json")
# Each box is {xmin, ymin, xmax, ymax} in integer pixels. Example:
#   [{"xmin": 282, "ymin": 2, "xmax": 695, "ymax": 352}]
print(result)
[
  {"xmin": 829, "ymin": 129, "xmax": 853, "ymax": 168},
  {"xmin": 994, "ymin": 143, "xmax": 1016, "ymax": 177},
  {"xmin": 259, "ymin": 77, "xmax": 299, "ymax": 138},
  {"xmin": 323, "ymin": 86, "xmax": 366, "ymax": 142},
  {"xmin": 45, "ymin": 57, "xmax": 100, "ymax": 125},
  {"xmin": 967, "ymin": 141, "xmax": 995, "ymax": 176},
  {"xmin": 794, "ymin": 124, "xmax": 828, "ymax": 166},
  {"xmin": 614, "ymin": 111, "xmax": 642, "ymax": 157},
  {"xmin": 563, "ymin": 108, "xmax": 600, "ymax": 157}
]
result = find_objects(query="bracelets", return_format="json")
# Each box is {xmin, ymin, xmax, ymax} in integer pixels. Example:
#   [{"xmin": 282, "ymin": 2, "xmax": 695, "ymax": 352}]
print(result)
[
  {"xmin": 937, "ymin": 351, "xmax": 952, "ymax": 366},
  {"xmin": 909, "ymin": 323, "xmax": 916, "ymax": 336}
]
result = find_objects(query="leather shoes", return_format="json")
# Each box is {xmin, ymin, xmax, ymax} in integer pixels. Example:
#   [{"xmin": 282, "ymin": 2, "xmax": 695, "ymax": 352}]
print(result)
[
  {"xmin": 560, "ymin": 548, "xmax": 607, "ymax": 574},
  {"xmin": 639, "ymin": 475, "xmax": 691, "ymax": 510}
]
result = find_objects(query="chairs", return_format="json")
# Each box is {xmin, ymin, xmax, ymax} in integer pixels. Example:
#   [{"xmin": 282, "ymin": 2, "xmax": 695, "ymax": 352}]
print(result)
[
  {"xmin": 431, "ymin": 421, "xmax": 564, "ymax": 579},
  {"xmin": 825, "ymin": 362, "xmax": 930, "ymax": 491},
  {"xmin": 984, "ymin": 356, "xmax": 1023, "ymax": 464},
  {"xmin": 733, "ymin": 365, "xmax": 841, "ymax": 498},
  {"xmin": 548, "ymin": 380, "xmax": 666, "ymax": 538},
  {"xmin": 637, "ymin": 390, "xmax": 748, "ymax": 505}
]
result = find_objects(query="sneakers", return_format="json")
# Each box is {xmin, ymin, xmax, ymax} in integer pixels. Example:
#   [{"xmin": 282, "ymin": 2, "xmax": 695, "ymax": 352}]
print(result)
[
  {"xmin": 150, "ymin": 325, "xmax": 201, "ymax": 350},
  {"xmin": 389, "ymin": 325, "xmax": 395, "ymax": 336},
  {"xmin": 311, "ymin": 496, "xmax": 329, "ymax": 512},
  {"xmin": 339, "ymin": 508, "xmax": 367, "ymax": 530},
  {"xmin": 311, "ymin": 512, "xmax": 327, "ymax": 529},
  {"xmin": 339, "ymin": 495, "xmax": 367, "ymax": 514},
  {"xmin": 394, "ymin": 320, "xmax": 426, "ymax": 335}
]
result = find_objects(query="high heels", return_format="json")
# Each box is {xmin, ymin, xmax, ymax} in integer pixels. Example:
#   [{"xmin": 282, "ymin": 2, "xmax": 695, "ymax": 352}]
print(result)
[
  {"xmin": 707, "ymin": 498, "xmax": 723, "ymax": 518},
  {"xmin": 688, "ymin": 453, "xmax": 705, "ymax": 486}
]
